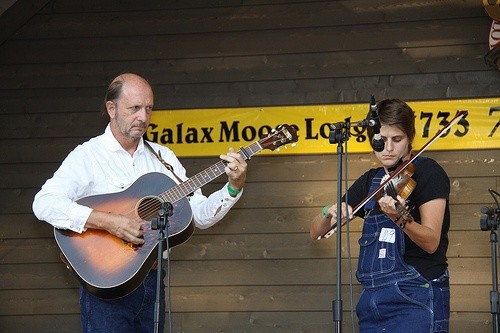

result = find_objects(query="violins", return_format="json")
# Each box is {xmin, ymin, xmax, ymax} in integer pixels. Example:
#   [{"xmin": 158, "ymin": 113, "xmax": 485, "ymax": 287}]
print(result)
[{"xmin": 373, "ymin": 160, "xmax": 416, "ymax": 224}]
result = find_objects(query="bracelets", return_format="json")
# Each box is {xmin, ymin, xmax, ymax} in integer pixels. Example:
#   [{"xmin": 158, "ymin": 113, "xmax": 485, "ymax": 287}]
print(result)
[
  {"xmin": 227, "ymin": 183, "xmax": 240, "ymax": 195},
  {"xmin": 321, "ymin": 205, "xmax": 331, "ymax": 220},
  {"xmin": 393, "ymin": 215, "xmax": 401, "ymax": 223},
  {"xmin": 400, "ymin": 220, "xmax": 406, "ymax": 231}
]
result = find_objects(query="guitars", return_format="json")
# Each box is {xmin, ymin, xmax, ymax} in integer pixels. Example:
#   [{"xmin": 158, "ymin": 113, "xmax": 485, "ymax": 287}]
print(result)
[{"xmin": 54, "ymin": 123, "xmax": 300, "ymax": 301}]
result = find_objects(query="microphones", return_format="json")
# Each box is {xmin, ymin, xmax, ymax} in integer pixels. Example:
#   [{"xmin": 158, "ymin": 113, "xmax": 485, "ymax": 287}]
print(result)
[
  {"xmin": 370, "ymin": 96, "xmax": 384, "ymax": 151},
  {"xmin": 160, "ymin": 202, "xmax": 173, "ymax": 212}
]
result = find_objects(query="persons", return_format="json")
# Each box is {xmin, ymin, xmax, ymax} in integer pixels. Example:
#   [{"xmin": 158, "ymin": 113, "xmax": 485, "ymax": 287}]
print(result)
[
  {"xmin": 32, "ymin": 73, "xmax": 248, "ymax": 333},
  {"xmin": 310, "ymin": 99, "xmax": 450, "ymax": 333}
]
